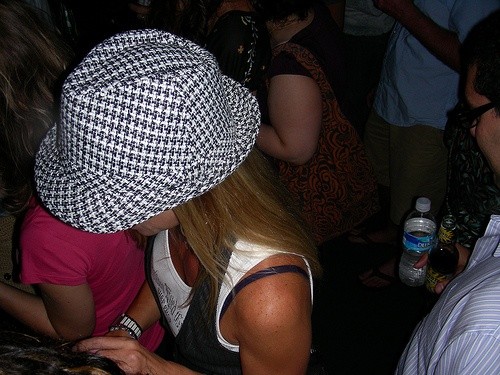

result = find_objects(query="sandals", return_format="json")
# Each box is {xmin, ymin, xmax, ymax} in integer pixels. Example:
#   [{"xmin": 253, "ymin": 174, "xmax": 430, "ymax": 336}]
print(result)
[
  {"xmin": 347, "ymin": 224, "xmax": 399, "ymax": 246},
  {"xmin": 358, "ymin": 260, "xmax": 398, "ymax": 289}
]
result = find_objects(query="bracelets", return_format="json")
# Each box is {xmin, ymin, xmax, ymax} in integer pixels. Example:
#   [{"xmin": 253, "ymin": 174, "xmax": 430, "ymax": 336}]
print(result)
[
  {"xmin": 110, "ymin": 324, "xmax": 136, "ymax": 340},
  {"xmin": 118, "ymin": 313, "xmax": 143, "ymax": 339}
]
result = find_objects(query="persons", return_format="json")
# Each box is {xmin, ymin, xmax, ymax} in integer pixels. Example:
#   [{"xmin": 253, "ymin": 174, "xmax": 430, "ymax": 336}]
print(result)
[
  {"xmin": 33, "ymin": 29, "xmax": 329, "ymax": 375},
  {"xmin": 21, "ymin": 0, "xmax": 500, "ymax": 287},
  {"xmin": 0, "ymin": 0, "xmax": 165, "ymax": 353},
  {"xmin": 395, "ymin": 7, "xmax": 500, "ymax": 375},
  {"xmin": 0, "ymin": 319, "xmax": 125, "ymax": 375}
]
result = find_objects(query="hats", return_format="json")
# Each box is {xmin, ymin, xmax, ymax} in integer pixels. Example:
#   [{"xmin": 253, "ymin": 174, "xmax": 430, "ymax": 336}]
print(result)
[{"xmin": 34, "ymin": 29, "xmax": 262, "ymax": 234}]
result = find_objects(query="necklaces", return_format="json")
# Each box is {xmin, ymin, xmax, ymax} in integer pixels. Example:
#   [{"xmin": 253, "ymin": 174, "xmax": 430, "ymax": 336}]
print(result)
[
  {"xmin": 176, "ymin": 225, "xmax": 188, "ymax": 246},
  {"xmin": 268, "ymin": 16, "xmax": 299, "ymax": 37}
]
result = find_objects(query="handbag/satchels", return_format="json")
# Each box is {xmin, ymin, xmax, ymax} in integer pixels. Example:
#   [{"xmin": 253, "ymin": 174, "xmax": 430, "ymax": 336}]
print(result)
[{"xmin": 264, "ymin": 42, "xmax": 382, "ymax": 244}]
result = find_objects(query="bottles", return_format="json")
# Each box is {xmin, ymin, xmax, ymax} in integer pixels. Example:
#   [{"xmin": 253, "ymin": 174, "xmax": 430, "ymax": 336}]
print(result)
[
  {"xmin": 423, "ymin": 215, "xmax": 459, "ymax": 301},
  {"xmin": 399, "ymin": 198, "xmax": 437, "ymax": 287}
]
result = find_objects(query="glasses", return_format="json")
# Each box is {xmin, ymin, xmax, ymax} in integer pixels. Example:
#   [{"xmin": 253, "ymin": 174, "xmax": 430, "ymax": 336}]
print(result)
[{"xmin": 450, "ymin": 99, "xmax": 496, "ymax": 129}]
